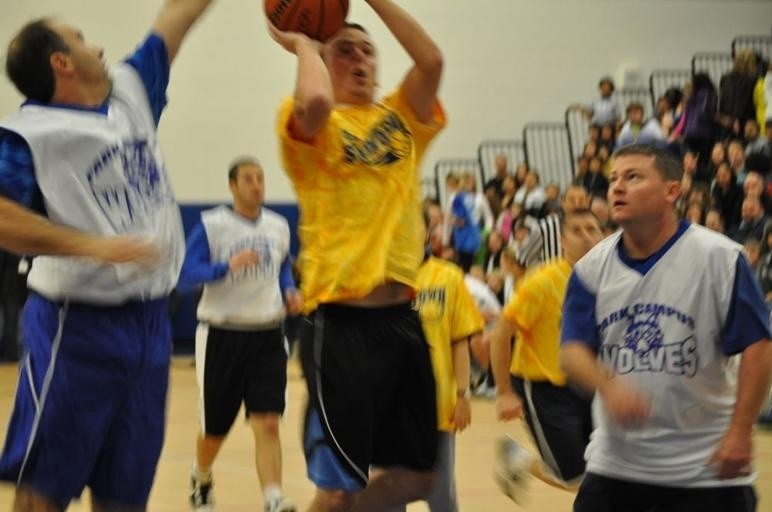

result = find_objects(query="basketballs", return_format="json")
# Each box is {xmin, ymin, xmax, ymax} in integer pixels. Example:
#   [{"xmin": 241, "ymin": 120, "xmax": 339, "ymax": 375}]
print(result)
[{"xmin": 262, "ymin": 0, "xmax": 348, "ymax": 44}]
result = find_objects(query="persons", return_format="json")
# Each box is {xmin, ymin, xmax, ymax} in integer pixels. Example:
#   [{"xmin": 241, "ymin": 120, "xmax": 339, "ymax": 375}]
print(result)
[
  {"xmin": 0, "ymin": 1, "xmax": 220, "ymax": 512},
  {"xmin": 172, "ymin": 154, "xmax": 305, "ymax": 511},
  {"xmin": 258, "ymin": 1, "xmax": 450, "ymax": 512}
]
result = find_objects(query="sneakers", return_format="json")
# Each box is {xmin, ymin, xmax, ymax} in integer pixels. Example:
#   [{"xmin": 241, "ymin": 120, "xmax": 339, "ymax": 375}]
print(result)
[
  {"xmin": 190, "ymin": 463, "xmax": 216, "ymax": 512},
  {"xmin": 264, "ymin": 495, "xmax": 298, "ymax": 512}
]
